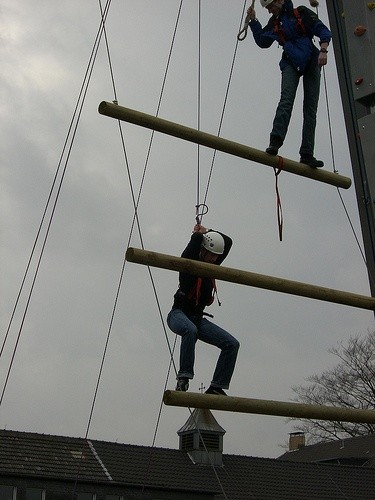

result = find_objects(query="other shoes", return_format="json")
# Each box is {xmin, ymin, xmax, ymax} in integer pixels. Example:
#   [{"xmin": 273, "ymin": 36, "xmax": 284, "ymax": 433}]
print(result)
[
  {"xmin": 299, "ymin": 157, "xmax": 324, "ymax": 167},
  {"xmin": 266, "ymin": 146, "xmax": 278, "ymax": 154},
  {"xmin": 204, "ymin": 387, "xmax": 228, "ymax": 396},
  {"xmin": 175, "ymin": 380, "xmax": 190, "ymax": 391}
]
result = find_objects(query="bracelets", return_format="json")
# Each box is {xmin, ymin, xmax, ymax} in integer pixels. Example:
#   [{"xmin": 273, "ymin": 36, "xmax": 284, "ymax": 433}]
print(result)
[{"xmin": 320, "ymin": 47, "xmax": 328, "ymax": 53}]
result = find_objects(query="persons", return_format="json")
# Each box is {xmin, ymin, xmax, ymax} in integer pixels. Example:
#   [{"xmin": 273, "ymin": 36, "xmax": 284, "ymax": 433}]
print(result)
[
  {"xmin": 167, "ymin": 225, "xmax": 241, "ymax": 396},
  {"xmin": 247, "ymin": 0, "xmax": 332, "ymax": 168}
]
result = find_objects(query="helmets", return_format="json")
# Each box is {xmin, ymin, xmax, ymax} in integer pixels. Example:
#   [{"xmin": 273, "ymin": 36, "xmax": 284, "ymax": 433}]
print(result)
[
  {"xmin": 259, "ymin": 0, "xmax": 272, "ymax": 7},
  {"xmin": 202, "ymin": 231, "xmax": 225, "ymax": 254}
]
